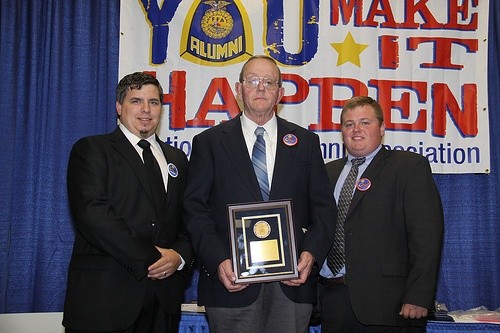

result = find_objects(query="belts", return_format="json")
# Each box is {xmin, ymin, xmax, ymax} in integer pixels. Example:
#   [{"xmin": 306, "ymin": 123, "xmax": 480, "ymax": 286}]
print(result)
[{"xmin": 318, "ymin": 275, "xmax": 347, "ymax": 284}]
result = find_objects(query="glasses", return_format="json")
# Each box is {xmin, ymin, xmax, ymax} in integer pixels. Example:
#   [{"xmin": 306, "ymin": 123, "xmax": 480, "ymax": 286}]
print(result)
[{"xmin": 240, "ymin": 77, "xmax": 280, "ymax": 90}]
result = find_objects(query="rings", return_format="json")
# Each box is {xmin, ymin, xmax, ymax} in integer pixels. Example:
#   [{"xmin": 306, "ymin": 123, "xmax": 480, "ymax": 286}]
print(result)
[{"xmin": 164, "ymin": 271, "xmax": 167, "ymax": 276}]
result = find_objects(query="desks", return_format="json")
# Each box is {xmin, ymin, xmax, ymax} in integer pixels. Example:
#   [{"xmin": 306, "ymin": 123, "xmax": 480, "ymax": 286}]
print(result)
[{"xmin": 179, "ymin": 303, "xmax": 500, "ymax": 333}]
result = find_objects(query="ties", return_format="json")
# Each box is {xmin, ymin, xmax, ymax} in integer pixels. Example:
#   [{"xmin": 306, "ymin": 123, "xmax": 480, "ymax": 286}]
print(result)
[
  {"xmin": 137, "ymin": 140, "xmax": 166, "ymax": 216},
  {"xmin": 327, "ymin": 157, "xmax": 366, "ymax": 276},
  {"xmin": 252, "ymin": 127, "xmax": 269, "ymax": 202}
]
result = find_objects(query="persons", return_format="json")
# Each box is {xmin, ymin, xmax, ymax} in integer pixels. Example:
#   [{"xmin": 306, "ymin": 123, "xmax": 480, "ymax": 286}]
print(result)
[
  {"xmin": 61, "ymin": 73, "xmax": 189, "ymax": 333},
  {"xmin": 183, "ymin": 55, "xmax": 336, "ymax": 333},
  {"xmin": 311, "ymin": 96, "xmax": 444, "ymax": 333}
]
